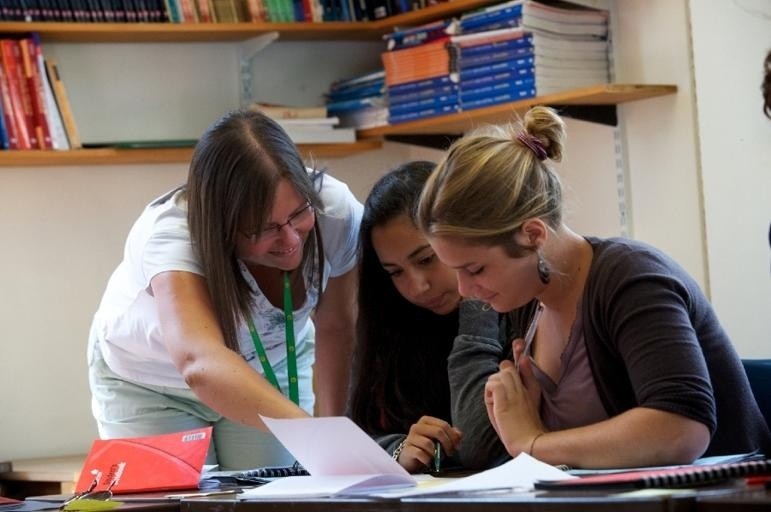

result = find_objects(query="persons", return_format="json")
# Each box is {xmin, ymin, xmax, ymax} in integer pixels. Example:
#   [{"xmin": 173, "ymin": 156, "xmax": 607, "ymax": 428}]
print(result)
[
  {"xmin": 414, "ymin": 107, "xmax": 767, "ymax": 467},
  {"xmin": 345, "ymin": 161, "xmax": 536, "ymax": 473},
  {"xmin": 87, "ymin": 110, "xmax": 365, "ymax": 472}
]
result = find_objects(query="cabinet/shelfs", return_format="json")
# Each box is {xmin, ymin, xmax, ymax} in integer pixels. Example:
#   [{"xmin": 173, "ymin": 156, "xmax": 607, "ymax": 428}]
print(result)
[{"xmin": 0, "ymin": 0, "xmax": 680, "ymax": 169}]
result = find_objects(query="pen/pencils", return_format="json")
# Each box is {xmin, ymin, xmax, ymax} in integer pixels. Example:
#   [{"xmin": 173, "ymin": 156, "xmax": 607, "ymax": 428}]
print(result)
[{"xmin": 434, "ymin": 440, "xmax": 442, "ymax": 476}]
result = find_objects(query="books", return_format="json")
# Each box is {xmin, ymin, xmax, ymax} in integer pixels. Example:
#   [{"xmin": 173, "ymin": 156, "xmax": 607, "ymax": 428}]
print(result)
[
  {"xmin": 73, "ymin": 425, "xmax": 220, "ymax": 493},
  {"xmin": 235, "ymin": 412, "xmax": 432, "ymax": 504},
  {"xmin": 535, "ymin": 461, "xmax": 770, "ymax": 491},
  {"xmin": 2, "ymin": 1, "xmax": 447, "ymax": 24},
  {"xmin": 0, "ymin": 37, "xmax": 84, "ymax": 153},
  {"xmin": 204, "ymin": 466, "xmax": 308, "ymax": 480},
  {"xmin": 250, "ymin": 1, "xmax": 613, "ymax": 145}
]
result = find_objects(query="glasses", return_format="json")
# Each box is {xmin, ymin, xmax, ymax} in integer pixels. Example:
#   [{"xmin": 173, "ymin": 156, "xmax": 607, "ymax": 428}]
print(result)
[{"xmin": 236, "ymin": 199, "xmax": 315, "ymax": 240}]
